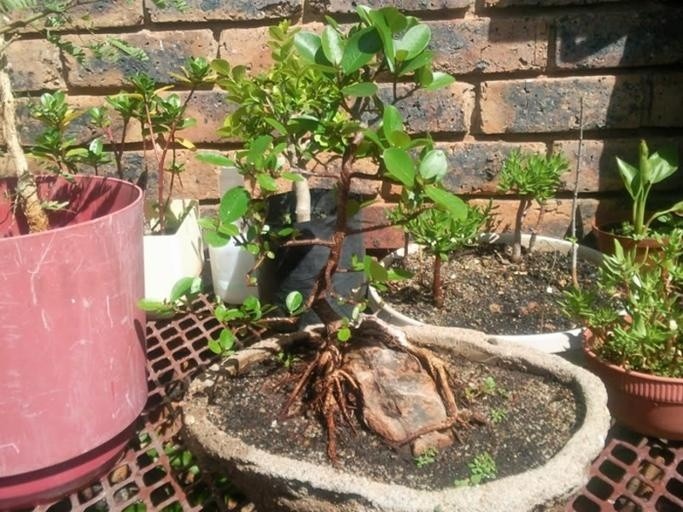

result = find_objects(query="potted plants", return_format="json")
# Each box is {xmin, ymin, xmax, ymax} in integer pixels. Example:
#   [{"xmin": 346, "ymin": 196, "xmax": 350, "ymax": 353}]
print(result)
[
  {"xmin": 0, "ymin": 0, "xmax": 149, "ymax": 510},
  {"xmin": 367, "ymin": 147, "xmax": 641, "ymax": 368},
  {"xmin": 207, "ymin": 18, "xmax": 369, "ymax": 327},
  {"xmin": 556, "ymin": 220, "xmax": 683, "ymax": 440},
  {"xmin": 185, "ymin": 6, "xmax": 611, "ymax": 512},
  {"xmin": 592, "ymin": 138, "xmax": 681, "ymax": 269},
  {"xmin": 30, "ymin": 58, "xmax": 214, "ymax": 304}
]
